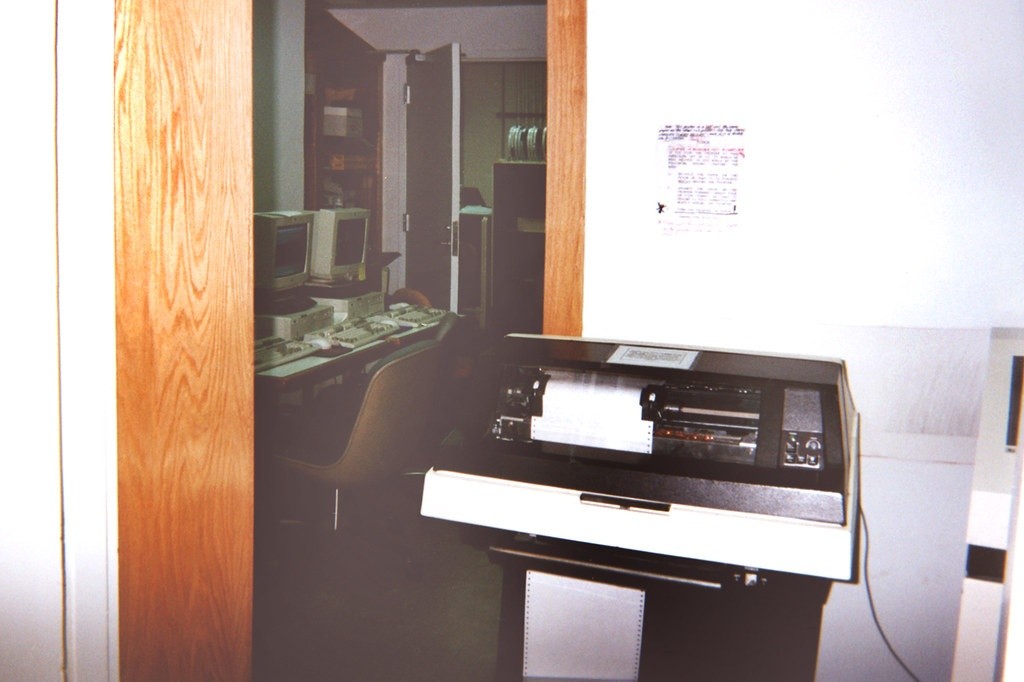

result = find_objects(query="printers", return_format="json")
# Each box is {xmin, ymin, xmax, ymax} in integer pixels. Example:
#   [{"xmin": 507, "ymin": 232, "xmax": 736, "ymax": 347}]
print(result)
[{"xmin": 381, "ymin": 335, "xmax": 860, "ymax": 596}]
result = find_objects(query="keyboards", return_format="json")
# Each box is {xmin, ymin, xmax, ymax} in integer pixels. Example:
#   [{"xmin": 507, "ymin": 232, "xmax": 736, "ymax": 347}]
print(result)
[
  {"xmin": 304, "ymin": 316, "xmax": 397, "ymax": 349},
  {"xmin": 254, "ymin": 334, "xmax": 322, "ymax": 373},
  {"xmin": 388, "ymin": 303, "xmax": 451, "ymax": 327}
]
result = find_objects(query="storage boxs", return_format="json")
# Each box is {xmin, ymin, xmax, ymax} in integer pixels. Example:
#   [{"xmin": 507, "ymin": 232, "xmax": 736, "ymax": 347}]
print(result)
[
  {"xmin": 255, "ymin": 305, "xmax": 334, "ymax": 341},
  {"xmin": 308, "ymin": 292, "xmax": 384, "ymax": 322}
]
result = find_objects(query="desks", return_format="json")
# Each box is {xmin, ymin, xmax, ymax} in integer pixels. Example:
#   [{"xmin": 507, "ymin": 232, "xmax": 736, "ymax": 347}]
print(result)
[{"xmin": 257, "ymin": 303, "xmax": 466, "ymax": 391}]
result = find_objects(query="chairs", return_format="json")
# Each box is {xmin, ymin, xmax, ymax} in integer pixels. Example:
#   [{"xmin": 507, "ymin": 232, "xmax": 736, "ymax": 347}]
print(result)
[
  {"xmin": 264, "ymin": 338, "xmax": 441, "ymax": 531},
  {"xmin": 309, "ymin": 310, "xmax": 465, "ymax": 475}
]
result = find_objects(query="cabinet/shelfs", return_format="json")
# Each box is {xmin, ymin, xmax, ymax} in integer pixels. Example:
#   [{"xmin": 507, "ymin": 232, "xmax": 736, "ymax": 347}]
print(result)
[
  {"xmin": 305, "ymin": 9, "xmax": 383, "ymax": 253},
  {"xmin": 488, "ymin": 530, "xmax": 834, "ymax": 682}
]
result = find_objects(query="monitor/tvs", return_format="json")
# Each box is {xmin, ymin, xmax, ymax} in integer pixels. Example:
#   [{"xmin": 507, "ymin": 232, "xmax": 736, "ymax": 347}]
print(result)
[
  {"xmin": 309, "ymin": 207, "xmax": 372, "ymax": 298},
  {"xmin": 254, "ymin": 210, "xmax": 333, "ymax": 315}
]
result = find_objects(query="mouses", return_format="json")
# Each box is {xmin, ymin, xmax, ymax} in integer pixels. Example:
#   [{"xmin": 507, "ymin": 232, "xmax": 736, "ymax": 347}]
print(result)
[
  {"xmin": 389, "ymin": 303, "xmax": 409, "ymax": 309},
  {"xmin": 376, "ymin": 318, "xmax": 401, "ymax": 330},
  {"xmin": 305, "ymin": 339, "xmax": 331, "ymax": 351}
]
